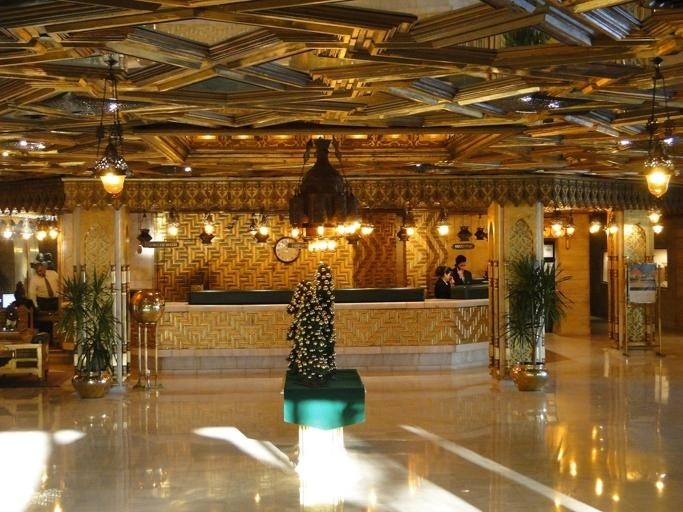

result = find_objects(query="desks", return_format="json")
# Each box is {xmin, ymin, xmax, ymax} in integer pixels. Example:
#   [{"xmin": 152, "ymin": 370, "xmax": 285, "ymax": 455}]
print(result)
[
  {"xmin": 0, "ymin": 331, "xmax": 49, "ymax": 382},
  {"xmin": 282, "ymin": 364, "xmax": 367, "ymax": 507}
]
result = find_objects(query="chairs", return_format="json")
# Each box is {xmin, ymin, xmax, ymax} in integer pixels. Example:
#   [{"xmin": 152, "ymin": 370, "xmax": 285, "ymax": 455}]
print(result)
[{"xmin": 8, "ymin": 304, "xmax": 33, "ymax": 329}]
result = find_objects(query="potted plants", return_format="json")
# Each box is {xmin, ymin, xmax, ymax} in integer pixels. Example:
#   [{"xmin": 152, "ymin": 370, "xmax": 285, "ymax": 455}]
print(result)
[
  {"xmin": 51, "ymin": 266, "xmax": 120, "ymax": 401},
  {"xmin": 498, "ymin": 253, "xmax": 572, "ymax": 394}
]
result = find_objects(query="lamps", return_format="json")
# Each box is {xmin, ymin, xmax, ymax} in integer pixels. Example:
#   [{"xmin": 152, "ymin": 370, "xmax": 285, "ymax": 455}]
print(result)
[
  {"xmin": 288, "ymin": 134, "xmax": 356, "ymax": 228},
  {"xmin": 544, "ymin": 197, "xmax": 666, "ymax": 241},
  {"xmin": 91, "ymin": 50, "xmax": 133, "ymax": 196},
  {"xmin": 642, "ymin": 56, "xmax": 679, "ymax": 198},
  {"xmin": 0, "ymin": 209, "xmax": 58, "ymax": 241},
  {"xmin": 122, "ymin": 196, "xmax": 490, "ymax": 253}
]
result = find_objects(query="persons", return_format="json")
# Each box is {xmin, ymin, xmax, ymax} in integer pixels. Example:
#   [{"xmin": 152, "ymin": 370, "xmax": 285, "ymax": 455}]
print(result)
[
  {"xmin": 8, "ymin": 279, "xmax": 34, "ymax": 315},
  {"xmin": 26, "ymin": 263, "xmax": 58, "ymax": 312},
  {"xmin": 449, "ymin": 254, "xmax": 473, "ymax": 285},
  {"xmin": 434, "ymin": 265, "xmax": 454, "ymax": 298}
]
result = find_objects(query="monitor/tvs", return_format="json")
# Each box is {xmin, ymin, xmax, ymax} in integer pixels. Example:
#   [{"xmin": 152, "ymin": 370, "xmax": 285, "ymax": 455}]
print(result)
[{"xmin": 2, "ymin": 293, "xmax": 16, "ymax": 308}]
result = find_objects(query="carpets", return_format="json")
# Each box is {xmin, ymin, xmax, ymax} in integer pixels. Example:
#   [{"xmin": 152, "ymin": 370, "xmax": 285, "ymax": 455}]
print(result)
[{"xmin": 0, "ymin": 371, "xmax": 72, "ymax": 387}]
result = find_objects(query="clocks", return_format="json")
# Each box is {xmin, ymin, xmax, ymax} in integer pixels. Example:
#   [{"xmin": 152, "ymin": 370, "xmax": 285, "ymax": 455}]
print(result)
[{"xmin": 273, "ymin": 236, "xmax": 301, "ymax": 264}]
